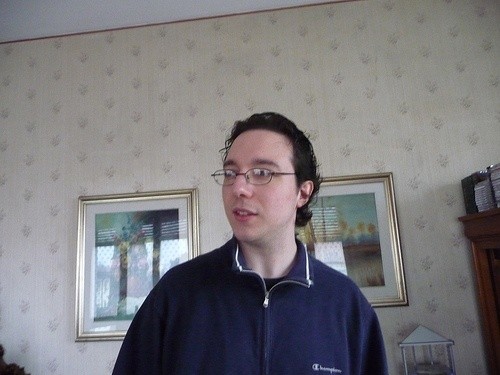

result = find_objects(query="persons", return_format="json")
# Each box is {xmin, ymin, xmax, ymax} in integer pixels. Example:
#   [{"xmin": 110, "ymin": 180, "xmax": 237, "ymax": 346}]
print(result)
[{"xmin": 112, "ymin": 112, "xmax": 390, "ymax": 375}]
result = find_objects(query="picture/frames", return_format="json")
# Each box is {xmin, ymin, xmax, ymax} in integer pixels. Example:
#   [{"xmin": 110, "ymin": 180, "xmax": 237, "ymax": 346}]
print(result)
[
  {"xmin": 296, "ymin": 171, "xmax": 410, "ymax": 308},
  {"xmin": 74, "ymin": 187, "xmax": 202, "ymax": 341}
]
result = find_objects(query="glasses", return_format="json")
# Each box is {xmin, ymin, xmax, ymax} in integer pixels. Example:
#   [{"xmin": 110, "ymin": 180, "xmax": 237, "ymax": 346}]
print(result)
[{"xmin": 211, "ymin": 169, "xmax": 297, "ymax": 185}]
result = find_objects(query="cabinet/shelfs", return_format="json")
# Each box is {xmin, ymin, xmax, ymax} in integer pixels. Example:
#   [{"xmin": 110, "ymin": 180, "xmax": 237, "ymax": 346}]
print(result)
[{"xmin": 457, "ymin": 205, "xmax": 500, "ymax": 375}]
